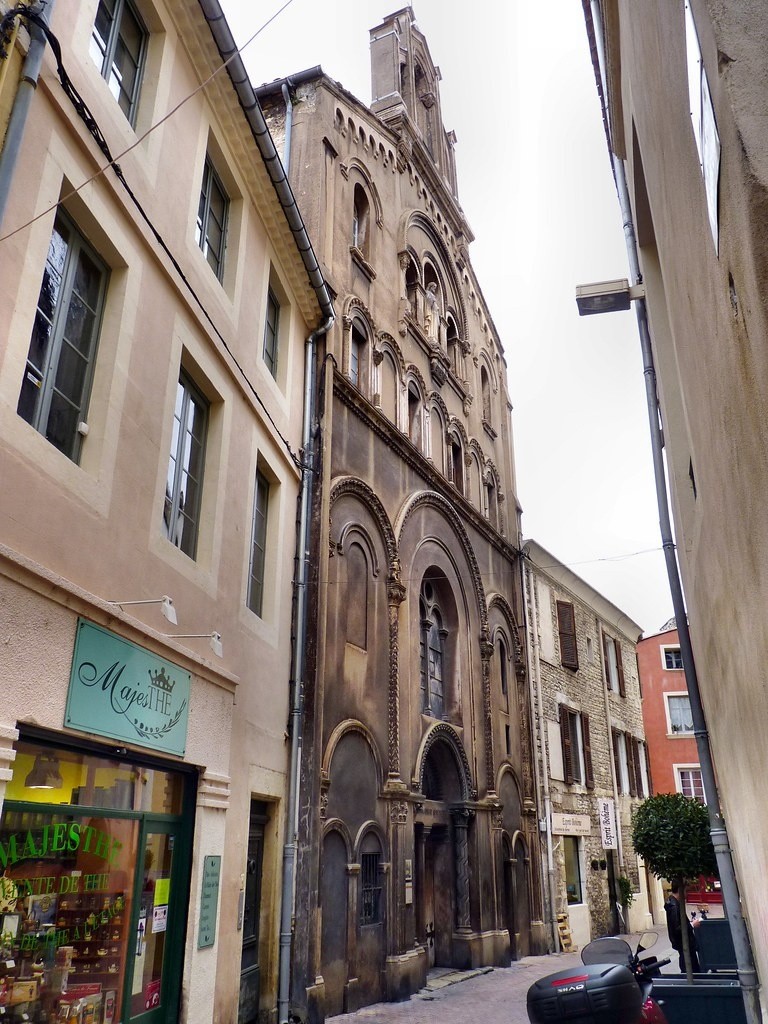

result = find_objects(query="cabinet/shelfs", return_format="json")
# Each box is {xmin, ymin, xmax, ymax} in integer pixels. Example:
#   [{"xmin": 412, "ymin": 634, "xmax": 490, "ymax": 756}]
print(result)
[{"xmin": 55, "ymin": 891, "xmax": 126, "ymax": 1008}]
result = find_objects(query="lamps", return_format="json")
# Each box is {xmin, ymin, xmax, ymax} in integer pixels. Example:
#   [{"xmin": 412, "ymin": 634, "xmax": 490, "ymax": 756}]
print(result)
[
  {"xmin": 109, "ymin": 595, "xmax": 177, "ymax": 626},
  {"xmin": 167, "ymin": 631, "xmax": 223, "ymax": 659},
  {"xmin": 576, "ymin": 278, "xmax": 645, "ymax": 316}
]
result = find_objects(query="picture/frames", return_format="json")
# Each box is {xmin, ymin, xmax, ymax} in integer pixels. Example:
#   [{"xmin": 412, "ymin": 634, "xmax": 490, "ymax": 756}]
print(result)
[{"xmin": 0, "ymin": 912, "xmax": 22, "ymax": 939}]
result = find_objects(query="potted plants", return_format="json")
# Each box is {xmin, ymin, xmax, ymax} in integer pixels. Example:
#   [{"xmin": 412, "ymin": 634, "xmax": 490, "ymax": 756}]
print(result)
[{"xmin": 631, "ymin": 792, "xmax": 747, "ymax": 1024}]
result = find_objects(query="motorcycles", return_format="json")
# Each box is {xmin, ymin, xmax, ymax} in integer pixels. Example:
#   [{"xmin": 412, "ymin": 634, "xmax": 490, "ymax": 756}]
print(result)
[{"xmin": 527, "ymin": 929, "xmax": 671, "ymax": 1024}]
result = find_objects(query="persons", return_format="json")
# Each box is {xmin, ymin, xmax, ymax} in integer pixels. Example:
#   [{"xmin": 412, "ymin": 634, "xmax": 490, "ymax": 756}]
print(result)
[{"xmin": 664, "ymin": 878, "xmax": 702, "ymax": 974}]
